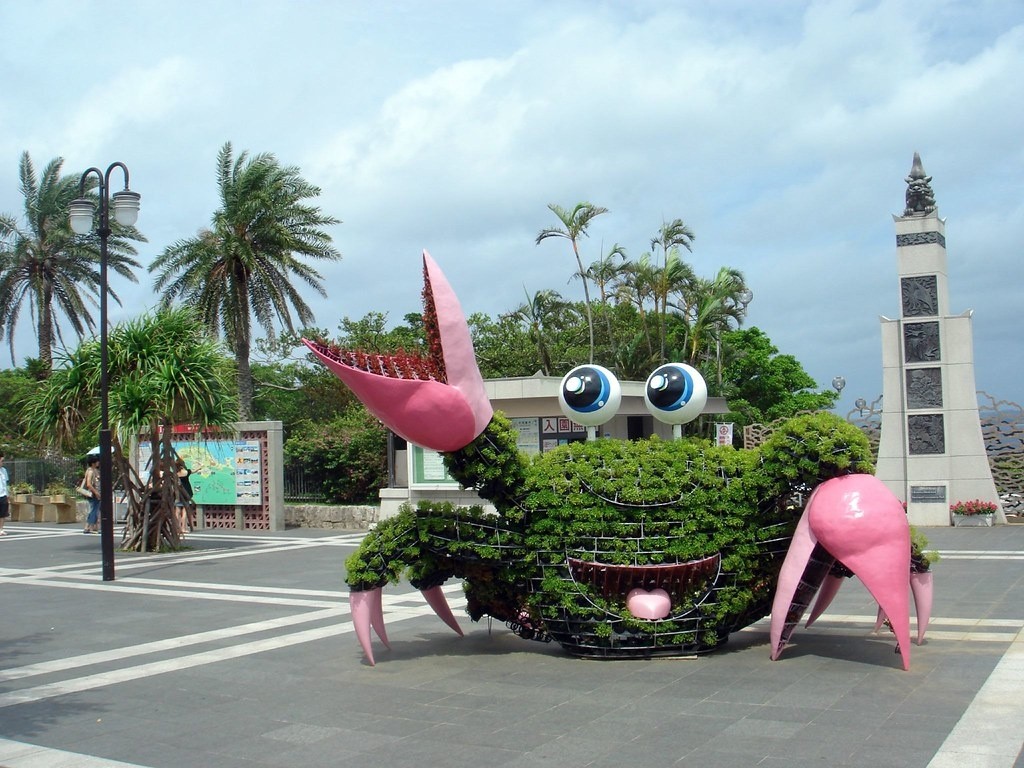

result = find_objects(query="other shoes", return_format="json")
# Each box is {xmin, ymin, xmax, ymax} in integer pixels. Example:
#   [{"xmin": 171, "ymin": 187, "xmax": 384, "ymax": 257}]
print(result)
[
  {"xmin": 83, "ymin": 528, "xmax": 101, "ymax": 534},
  {"xmin": 0, "ymin": 529, "xmax": 8, "ymax": 536}
]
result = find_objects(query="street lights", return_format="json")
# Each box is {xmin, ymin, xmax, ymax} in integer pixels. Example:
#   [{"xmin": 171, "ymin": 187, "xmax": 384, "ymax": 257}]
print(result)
[{"xmin": 68, "ymin": 162, "xmax": 141, "ymax": 581}]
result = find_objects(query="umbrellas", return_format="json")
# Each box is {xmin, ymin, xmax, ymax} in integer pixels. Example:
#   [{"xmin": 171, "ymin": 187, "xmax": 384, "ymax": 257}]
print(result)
[{"xmin": 86, "ymin": 446, "xmax": 115, "ymax": 455}]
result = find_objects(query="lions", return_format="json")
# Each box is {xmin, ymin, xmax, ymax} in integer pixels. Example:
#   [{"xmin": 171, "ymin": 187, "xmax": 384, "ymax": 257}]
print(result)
[{"xmin": 903, "ymin": 175, "xmax": 936, "ymax": 216}]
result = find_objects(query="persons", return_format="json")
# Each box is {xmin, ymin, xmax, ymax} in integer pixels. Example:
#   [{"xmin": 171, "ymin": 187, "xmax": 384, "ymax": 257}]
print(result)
[
  {"xmin": 0, "ymin": 450, "xmax": 11, "ymax": 536},
  {"xmin": 149, "ymin": 458, "xmax": 194, "ymax": 533},
  {"xmin": 83, "ymin": 457, "xmax": 101, "ymax": 534}
]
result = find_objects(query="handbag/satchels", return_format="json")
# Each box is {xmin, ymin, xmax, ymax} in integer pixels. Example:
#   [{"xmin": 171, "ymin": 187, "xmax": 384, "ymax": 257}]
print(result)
[{"xmin": 76, "ymin": 468, "xmax": 95, "ymax": 497}]
